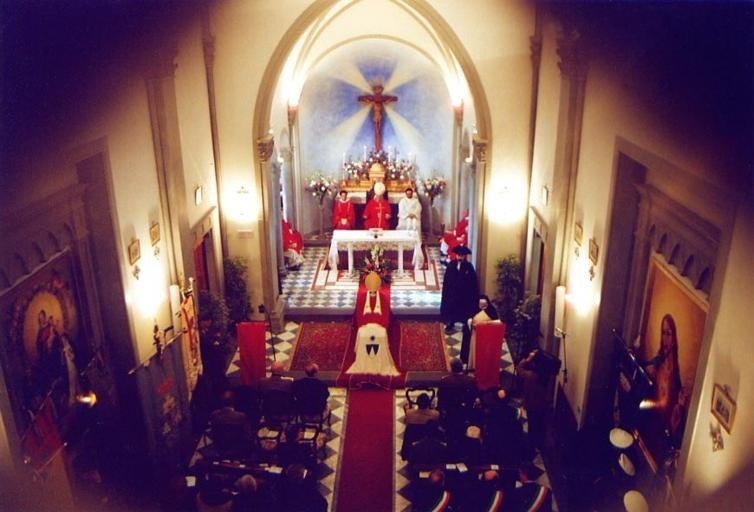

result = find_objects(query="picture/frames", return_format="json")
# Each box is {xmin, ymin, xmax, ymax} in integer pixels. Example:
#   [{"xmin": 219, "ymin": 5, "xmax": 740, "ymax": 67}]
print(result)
[
  {"xmin": 710, "ymin": 383, "xmax": 736, "ymax": 434},
  {"xmin": 624, "ymin": 247, "xmax": 709, "ymax": 490},
  {"xmin": 0, "ymin": 244, "xmax": 111, "ymax": 483},
  {"xmin": 574, "ymin": 222, "xmax": 583, "ymax": 246},
  {"xmin": 150, "ymin": 223, "xmax": 160, "ymax": 246},
  {"xmin": 542, "ymin": 186, "xmax": 548, "ymax": 206},
  {"xmin": 588, "ymin": 239, "xmax": 598, "ymax": 265},
  {"xmin": 129, "ymin": 239, "xmax": 140, "ymax": 265}
]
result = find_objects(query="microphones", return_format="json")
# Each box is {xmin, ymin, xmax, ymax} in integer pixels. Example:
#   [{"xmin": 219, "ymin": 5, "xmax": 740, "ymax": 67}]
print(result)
[
  {"xmin": 555, "ymin": 328, "xmax": 567, "ymax": 336},
  {"xmin": 516, "ymin": 309, "xmax": 531, "ymax": 320}
]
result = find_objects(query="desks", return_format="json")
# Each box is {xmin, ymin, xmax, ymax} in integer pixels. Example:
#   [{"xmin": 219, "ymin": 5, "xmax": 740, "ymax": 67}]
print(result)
[{"xmin": 324, "ymin": 229, "xmax": 429, "ymax": 269}]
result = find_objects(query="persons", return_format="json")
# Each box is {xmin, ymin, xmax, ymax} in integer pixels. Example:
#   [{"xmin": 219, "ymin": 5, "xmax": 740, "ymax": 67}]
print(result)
[
  {"xmin": 436, "ymin": 208, "xmax": 471, "ymax": 260},
  {"xmin": 34, "ymin": 305, "xmax": 62, "ymax": 370},
  {"xmin": 186, "ymin": 342, "xmax": 331, "ymax": 511},
  {"xmin": 461, "ymin": 295, "xmax": 498, "ymax": 363},
  {"xmin": 281, "ymin": 214, "xmax": 307, "ymax": 270},
  {"xmin": 198, "ymin": 310, "xmax": 227, "ymax": 376},
  {"xmin": 642, "ymin": 312, "xmax": 695, "ymax": 435},
  {"xmin": 333, "ymin": 190, "xmax": 358, "ymax": 232},
  {"xmin": 363, "ymin": 181, "xmax": 394, "ymax": 231},
  {"xmin": 403, "ymin": 353, "xmax": 555, "ymax": 511},
  {"xmin": 397, "ymin": 185, "xmax": 422, "ymax": 251},
  {"xmin": 363, "ymin": 96, "xmax": 393, "ymax": 152},
  {"xmin": 438, "ymin": 247, "xmax": 481, "ymax": 336}
]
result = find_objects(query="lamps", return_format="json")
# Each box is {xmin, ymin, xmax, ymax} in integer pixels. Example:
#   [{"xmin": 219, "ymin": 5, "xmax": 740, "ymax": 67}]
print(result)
[
  {"xmin": 553, "ymin": 285, "xmax": 566, "ymax": 338},
  {"xmin": 288, "ymin": 103, "xmax": 299, "ymax": 128},
  {"xmin": 453, "ymin": 106, "xmax": 463, "ymax": 127},
  {"xmin": 169, "ymin": 285, "xmax": 182, "ymax": 335}
]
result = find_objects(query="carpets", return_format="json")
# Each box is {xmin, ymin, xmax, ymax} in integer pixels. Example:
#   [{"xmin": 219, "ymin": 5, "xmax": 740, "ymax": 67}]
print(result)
[
  {"xmin": 289, "ymin": 319, "xmax": 452, "ymax": 375},
  {"xmin": 311, "ymin": 259, "xmax": 440, "ymax": 290}
]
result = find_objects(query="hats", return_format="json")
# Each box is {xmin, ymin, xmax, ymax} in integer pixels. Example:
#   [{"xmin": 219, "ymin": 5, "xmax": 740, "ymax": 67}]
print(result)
[{"xmin": 453, "ymin": 246, "xmax": 472, "ymax": 255}]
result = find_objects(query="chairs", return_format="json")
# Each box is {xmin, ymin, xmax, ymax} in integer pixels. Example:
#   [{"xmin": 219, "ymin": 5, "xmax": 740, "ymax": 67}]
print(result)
[
  {"xmin": 187, "ymin": 377, "xmax": 334, "ymax": 512},
  {"xmin": 403, "ymin": 376, "xmax": 553, "ymax": 512}
]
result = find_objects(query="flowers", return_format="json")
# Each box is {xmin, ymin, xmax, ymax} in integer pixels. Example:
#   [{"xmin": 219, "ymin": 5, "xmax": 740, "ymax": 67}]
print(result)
[
  {"xmin": 303, "ymin": 171, "xmax": 339, "ymax": 205},
  {"xmin": 419, "ymin": 177, "xmax": 446, "ymax": 206}
]
